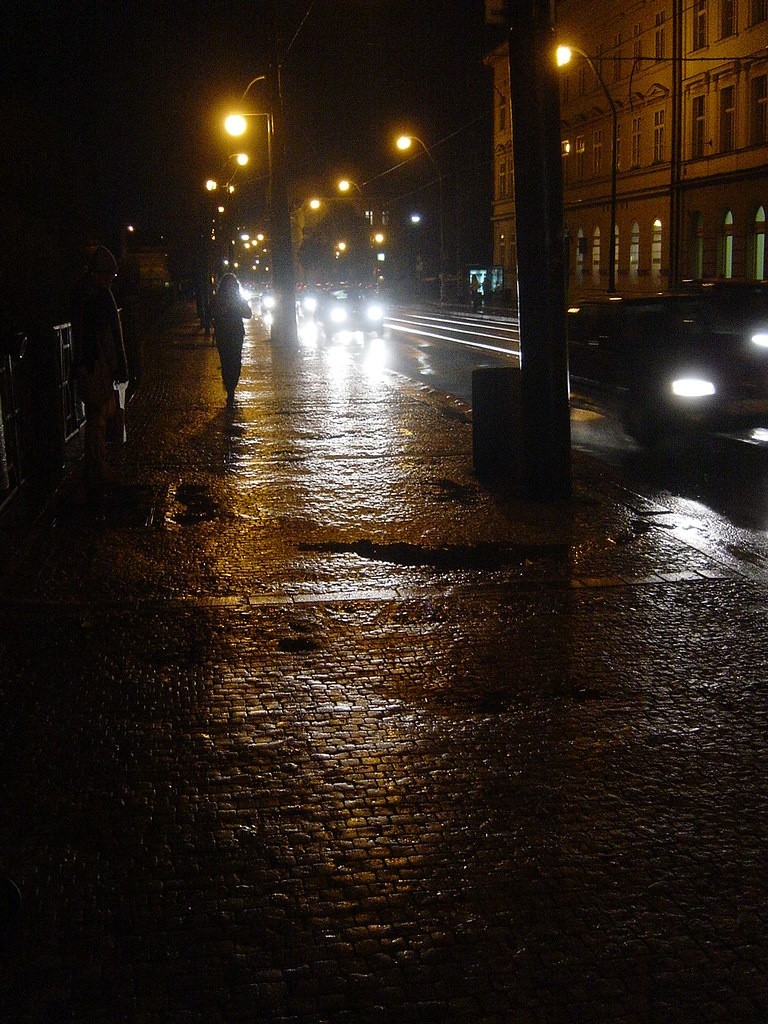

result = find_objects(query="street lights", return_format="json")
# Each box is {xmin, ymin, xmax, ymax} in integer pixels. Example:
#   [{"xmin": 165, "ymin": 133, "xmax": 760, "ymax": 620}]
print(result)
[
  {"xmin": 397, "ymin": 134, "xmax": 447, "ymax": 303},
  {"xmin": 229, "ymin": 111, "xmax": 296, "ymax": 339},
  {"xmin": 314, "ymin": 198, "xmax": 339, "ymax": 271},
  {"xmin": 556, "ymin": 44, "xmax": 616, "ymax": 295},
  {"xmin": 339, "ymin": 179, "xmax": 379, "ymax": 293}
]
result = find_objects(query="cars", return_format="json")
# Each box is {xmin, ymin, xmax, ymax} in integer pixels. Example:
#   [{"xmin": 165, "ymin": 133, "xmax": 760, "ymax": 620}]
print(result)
[
  {"xmin": 567, "ymin": 290, "xmax": 768, "ymax": 444},
  {"xmin": 669, "ymin": 279, "xmax": 768, "ymax": 293}
]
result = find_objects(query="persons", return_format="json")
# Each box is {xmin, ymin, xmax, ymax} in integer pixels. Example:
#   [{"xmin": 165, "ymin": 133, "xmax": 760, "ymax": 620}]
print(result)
[
  {"xmin": 209, "ymin": 273, "xmax": 253, "ymax": 403},
  {"xmin": 72, "ymin": 246, "xmax": 130, "ymax": 482}
]
